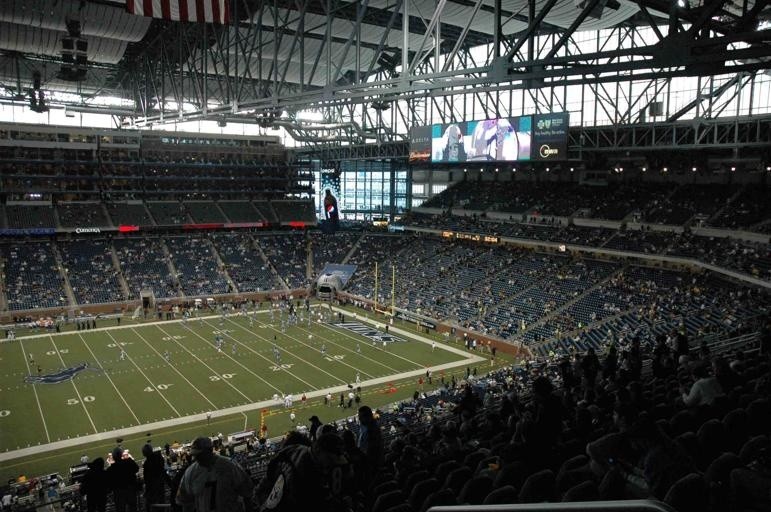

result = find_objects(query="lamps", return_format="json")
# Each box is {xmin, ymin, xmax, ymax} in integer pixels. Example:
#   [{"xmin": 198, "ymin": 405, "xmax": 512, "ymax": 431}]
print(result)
[
  {"xmin": 375, "ymin": 53, "xmax": 400, "ymax": 78},
  {"xmin": 369, "ymin": 100, "xmax": 390, "ymax": 111}
]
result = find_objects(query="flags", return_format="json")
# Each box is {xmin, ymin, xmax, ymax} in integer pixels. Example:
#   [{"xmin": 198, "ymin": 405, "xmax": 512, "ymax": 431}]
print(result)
[{"xmin": 125, "ymin": 0, "xmax": 230, "ymax": 26}]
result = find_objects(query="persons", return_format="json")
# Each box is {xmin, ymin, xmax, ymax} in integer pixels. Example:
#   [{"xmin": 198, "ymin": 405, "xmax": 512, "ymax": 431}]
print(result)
[{"xmin": 2, "ymin": 138, "xmax": 771, "ymax": 511}]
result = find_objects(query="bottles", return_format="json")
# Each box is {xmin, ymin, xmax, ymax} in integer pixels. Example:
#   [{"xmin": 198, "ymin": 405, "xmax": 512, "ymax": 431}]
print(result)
[
  {"xmin": 324, "ymin": 188, "xmax": 339, "ymax": 224},
  {"xmin": 627, "ymin": 472, "xmax": 649, "ymax": 489}
]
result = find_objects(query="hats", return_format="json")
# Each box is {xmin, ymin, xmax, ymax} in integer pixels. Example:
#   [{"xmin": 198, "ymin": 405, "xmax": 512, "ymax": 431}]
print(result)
[
  {"xmin": 189, "ymin": 437, "xmax": 211, "ymax": 454},
  {"xmin": 318, "ymin": 432, "xmax": 349, "ymax": 465},
  {"xmin": 309, "ymin": 416, "xmax": 317, "ymax": 421}
]
